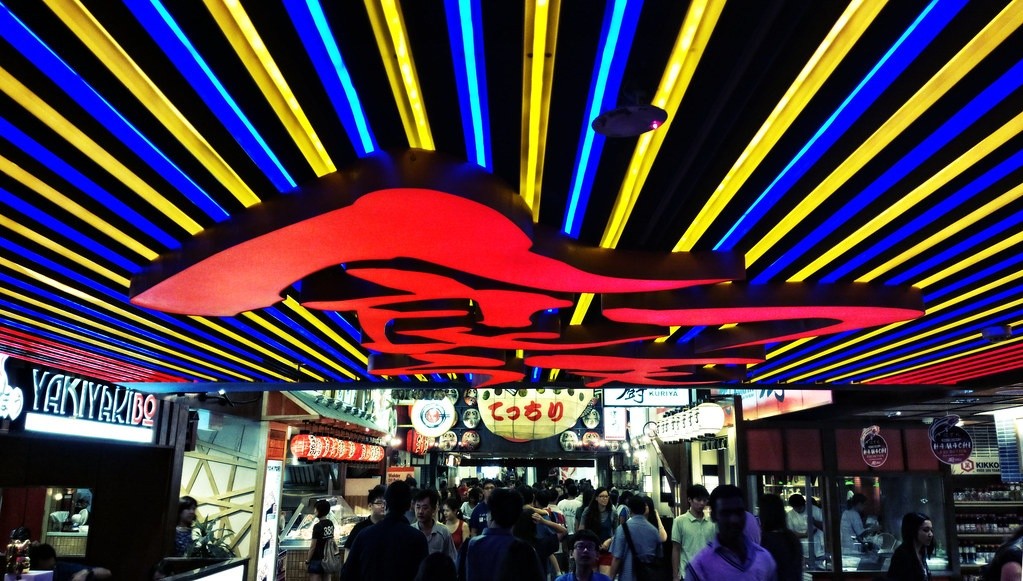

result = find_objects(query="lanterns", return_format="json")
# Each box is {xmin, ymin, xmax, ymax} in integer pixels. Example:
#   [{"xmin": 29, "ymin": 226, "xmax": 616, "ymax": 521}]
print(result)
[
  {"xmin": 290, "ymin": 434, "xmax": 384, "ymax": 462},
  {"xmin": 559, "ymin": 395, "xmax": 600, "ymax": 453},
  {"xmin": 444, "ymin": 389, "xmax": 459, "ymax": 405},
  {"xmin": 464, "ymin": 389, "xmax": 477, "ymax": 406},
  {"xmin": 657, "ymin": 402, "xmax": 724, "ymax": 444},
  {"xmin": 463, "ymin": 409, "xmax": 480, "ymax": 429},
  {"xmin": 452, "ymin": 411, "xmax": 458, "ymax": 426},
  {"xmin": 407, "ymin": 430, "xmax": 479, "ymax": 454}
]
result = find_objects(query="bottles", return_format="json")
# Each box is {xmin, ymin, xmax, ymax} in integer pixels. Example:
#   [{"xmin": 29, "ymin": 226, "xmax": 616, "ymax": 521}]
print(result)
[
  {"xmin": 936, "ymin": 540, "xmax": 943, "ymax": 557},
  {"xmin": 955, "ymin": 482, "xmax": 1021, "ymax": 581}
]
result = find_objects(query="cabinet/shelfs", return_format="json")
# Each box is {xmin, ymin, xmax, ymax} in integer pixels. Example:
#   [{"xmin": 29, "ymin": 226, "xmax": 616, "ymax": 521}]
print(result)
[{"xmin": 950, "ymin": 500, "xmax": 1023, "ymax": 581}]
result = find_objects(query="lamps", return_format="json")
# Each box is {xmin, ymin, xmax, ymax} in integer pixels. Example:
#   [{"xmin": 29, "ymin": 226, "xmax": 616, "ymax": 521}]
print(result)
[{"xmin": 504, "ymin": 388, "xmax": 518, "ymax": 396}]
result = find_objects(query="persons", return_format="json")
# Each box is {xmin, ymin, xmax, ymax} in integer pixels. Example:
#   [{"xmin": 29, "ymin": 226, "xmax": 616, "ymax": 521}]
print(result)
[
  {"xmin": 985, "ymin": 526, "xmax": 1023, "ymax": 581},
  {"xmin": 405, "ymin": 477, "xmax": 668, "ymax": 581},
  {"xmin": 756, "ymin": 494, "xmax": 803, "ymax": 581},
  {"xmin": 343, "ymin": 485, "xmax": 387, "ymax": 563},
  {"xmin": 466, "ymin": 488, "xmax": 544, "ymax": 581},
  {"xmin": 31, "ymin": 544, "xmax": 112, "ymax": 581},
  {"xmin": 303, "ymin": 500, "xmax": 334, "ymax": 581},
  {"xmin": 685, "ymin": 484, "xmax": 777, "ymax": 581},
  {"xmin": 887, "ymin": 511, "xmax": 938, "ymax": 581},
  {"xmin": 418, "ymin": 550, "xmax": 457, "ymax": 581},
  {"xmin": 745, "ymin": 493, "xmax": 877, "ymax": 556},
  {"xmin": 340, "ymin": 480, "xmax": 430, "ymax": 581},
  {"xmin": 176, "ymin": 496, "xmax": 197, "ymax": 553},
  {"xmin": 671, "ymin": 484, "xmax": 716, "ymax": 581}
]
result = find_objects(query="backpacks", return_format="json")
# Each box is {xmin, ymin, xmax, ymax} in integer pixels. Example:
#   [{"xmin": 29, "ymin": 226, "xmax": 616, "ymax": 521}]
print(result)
[{"xmin": 317, "ymin": 522, "xmax": 341, "ymax": 575}]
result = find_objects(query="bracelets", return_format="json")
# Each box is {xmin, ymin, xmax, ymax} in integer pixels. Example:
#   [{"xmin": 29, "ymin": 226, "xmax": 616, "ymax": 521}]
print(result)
[
  {"xmin": 305, "ymin": 560, "xmax": 310, "ymax": 564},
  {"xmin": 88, "ymin": 568, "xmax": 94, "ymax": 574}
]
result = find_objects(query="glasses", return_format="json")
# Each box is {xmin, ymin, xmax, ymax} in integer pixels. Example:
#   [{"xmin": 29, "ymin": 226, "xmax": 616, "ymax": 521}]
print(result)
[
  {"xmin": 484, "ymin": 486, "xmax": 495, "ymax": 491},
  {"xmin": 371, "ymin": 500, "xmax": 385, "ymax": 507},
  {"xmin": 596, "ymin": 495, "xmax": 609, "ymax": 499},
  {"xmin": 574, "ymin": 543, "xmax": 597, "ymax": 551}
]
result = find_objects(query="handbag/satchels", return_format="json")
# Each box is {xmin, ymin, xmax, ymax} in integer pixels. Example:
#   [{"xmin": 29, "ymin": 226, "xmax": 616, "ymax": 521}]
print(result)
[
  {"xmin": 654, "ymin": 509, "xmax": 667, "ymax": 542},
  {"xmin": 632, "ymin": 554, "xmax": 664, "ymax": 581}
]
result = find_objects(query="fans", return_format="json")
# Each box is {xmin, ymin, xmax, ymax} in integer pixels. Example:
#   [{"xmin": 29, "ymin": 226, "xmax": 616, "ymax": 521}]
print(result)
[{"xmin": 71, "ymin": 509, "xmax": 88, "ymax": 532}]
